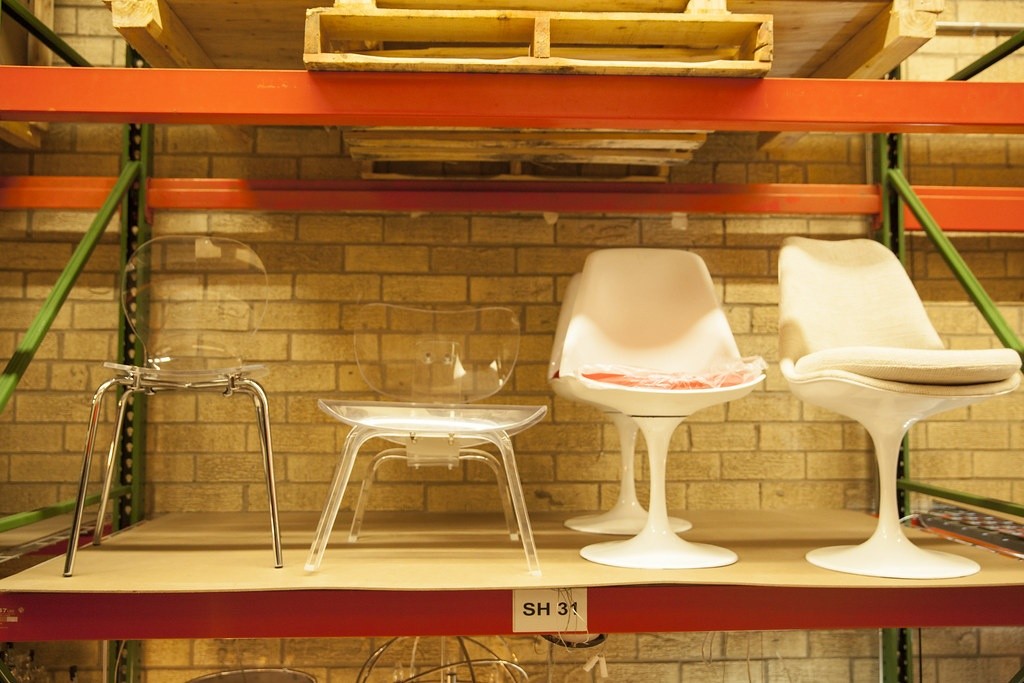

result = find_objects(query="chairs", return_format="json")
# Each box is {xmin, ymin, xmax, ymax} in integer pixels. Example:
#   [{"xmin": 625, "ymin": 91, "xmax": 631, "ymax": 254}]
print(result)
[
  {"xmin": 305, "ymin": 303, "xmax": 550, "ymax": 588},
  {"xmin": 549, "ymin": 246, "xmax": 767, "ymax": 574},
  {"xmin": 766, "ymin": 232, "xmax": 1022, "ymax": 579},
  {"xmin": 60, "ymin": 235, "xmax": 283, "ymax": 579}
]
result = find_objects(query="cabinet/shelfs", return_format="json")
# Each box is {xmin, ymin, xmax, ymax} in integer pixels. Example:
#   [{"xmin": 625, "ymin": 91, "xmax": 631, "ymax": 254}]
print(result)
[{"xmin": 0, "ymin": 0, "xmax": 1024, "ymax": 683}]
